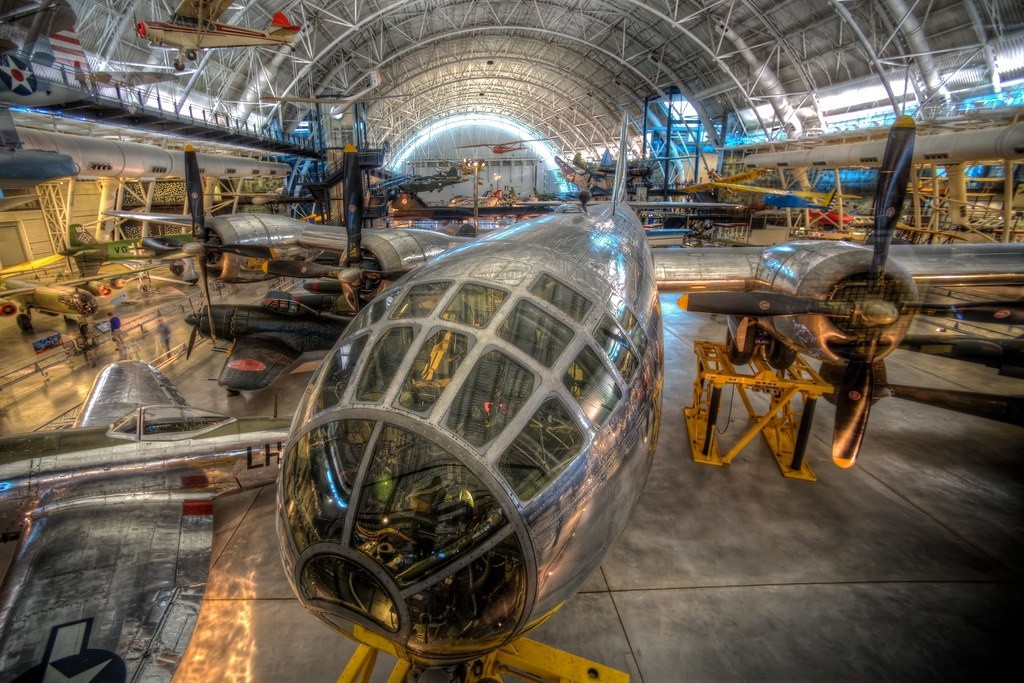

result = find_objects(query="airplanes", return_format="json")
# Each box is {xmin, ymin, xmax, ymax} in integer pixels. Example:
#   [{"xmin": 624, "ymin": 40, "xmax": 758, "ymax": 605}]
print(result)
[
  {"xmin": 0, "ymin": 86, "xmax": 1023, "ymax": 683},
  {"xmin": 133, "ymin": 3, "xmax": 302, "ymax": 72}
]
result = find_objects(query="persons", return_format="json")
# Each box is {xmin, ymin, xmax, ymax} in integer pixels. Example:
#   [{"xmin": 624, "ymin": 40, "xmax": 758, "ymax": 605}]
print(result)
[
  {"xmin": 107, "ymin": 312, "xmax": 125, "ymax": 351},
  {"xmin": 74, "ymin": 60, "xmax": 91, "ymax": 95},
  {"xmin": 383, "ymin": 217, "xmax": 390, "ymax": 228},
  {"xmin": 157, "ymin": 317, "xmax": 172, "ymax": 359}
]
